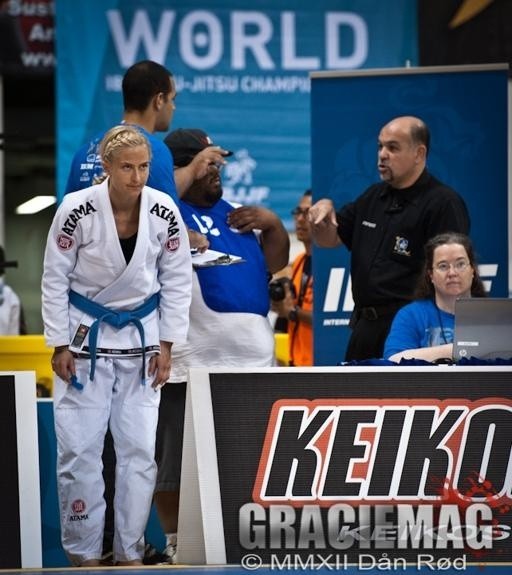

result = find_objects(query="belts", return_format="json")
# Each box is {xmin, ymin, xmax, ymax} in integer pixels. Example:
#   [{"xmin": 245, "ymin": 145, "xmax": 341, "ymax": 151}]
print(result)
[{"xmin": 357, "ymin": 303, "xmax": 399, "ymax": 321}]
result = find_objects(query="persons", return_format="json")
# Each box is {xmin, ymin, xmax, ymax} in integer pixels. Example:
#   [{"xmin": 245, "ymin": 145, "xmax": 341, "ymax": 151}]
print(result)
[
  {"xmin": 68, "ymin": 61, "xmax": 179, "ymax": 565},
  {"xmin": 40, "ymin": 127, "xmax": 195, "ymax": 568},
  {"xmin": 0, "ymin": 250, "xmax": 21, "ymax": 336},
  {"xmin": 154, "ymin": 129, "xmax": 290, "ymax": 564},
  {"xmin": 384, "ymin": 233, "xmax": 486, "ymax": 365},
  {"xmin": 270, "ymin": 189, "xmax": 316, "ymax": 366},
  {"xmin": 307, "ymin": 115, "xmax": 468, "ymax": 361}
]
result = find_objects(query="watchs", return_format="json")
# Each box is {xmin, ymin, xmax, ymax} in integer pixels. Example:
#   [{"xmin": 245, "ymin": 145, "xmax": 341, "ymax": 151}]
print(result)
[{"xmin": 288, "ymin": 304, "xmax": 299, "ymax": 320}]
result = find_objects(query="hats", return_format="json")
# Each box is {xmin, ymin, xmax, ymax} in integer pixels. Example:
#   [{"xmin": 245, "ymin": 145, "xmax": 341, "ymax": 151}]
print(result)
[{"xmin": 163, "ymin": 127, "xmax": 234, "ymax": 169}]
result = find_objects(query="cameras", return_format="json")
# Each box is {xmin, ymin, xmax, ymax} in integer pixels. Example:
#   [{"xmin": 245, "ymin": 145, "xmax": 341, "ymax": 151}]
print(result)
[{"xmin": 268, "ymin": 277, "xmax": 297, "ymax": 301}]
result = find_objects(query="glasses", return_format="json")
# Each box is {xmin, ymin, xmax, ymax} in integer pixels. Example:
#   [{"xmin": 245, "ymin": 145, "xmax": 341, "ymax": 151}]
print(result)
[
  {"xmin": 291, "ymin": 206, "xmax": 310, "ymax": 217},
  {"xmin": 431, "ymin": 260, "xmax": 471, "ymax": 273}
]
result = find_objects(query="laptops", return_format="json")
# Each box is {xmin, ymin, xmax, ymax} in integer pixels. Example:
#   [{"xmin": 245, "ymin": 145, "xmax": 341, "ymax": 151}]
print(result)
[{"xmin": 451, "ymin": 297, "xmax": 512, "ymax": 362}]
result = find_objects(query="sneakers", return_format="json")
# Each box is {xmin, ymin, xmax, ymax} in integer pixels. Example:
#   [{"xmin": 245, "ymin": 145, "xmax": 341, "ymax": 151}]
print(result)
[{"xmin": 143, "ymin": 544, "xmax": 170, "ymax": 565}]
